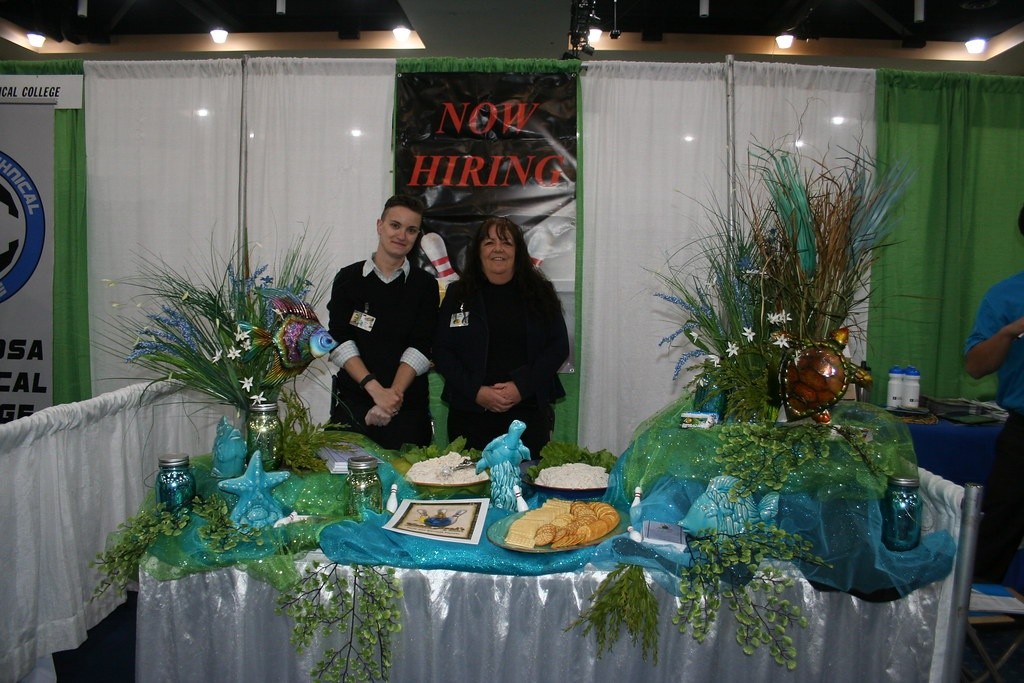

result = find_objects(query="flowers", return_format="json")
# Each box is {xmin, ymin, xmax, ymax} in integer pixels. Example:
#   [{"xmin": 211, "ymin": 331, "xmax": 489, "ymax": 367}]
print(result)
[
  {"xmin": 88, "ymin": 217, "xmax": 358, "ymax": 438},
  {"xmin": 651, "ymin": 148, "xmax": 922, "ymax": 422}
]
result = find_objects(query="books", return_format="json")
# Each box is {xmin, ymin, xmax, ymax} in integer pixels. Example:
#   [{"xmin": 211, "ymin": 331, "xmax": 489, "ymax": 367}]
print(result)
[{"xmin": 316, "ymin": 442, "xmax": 385, "ymax": 474}]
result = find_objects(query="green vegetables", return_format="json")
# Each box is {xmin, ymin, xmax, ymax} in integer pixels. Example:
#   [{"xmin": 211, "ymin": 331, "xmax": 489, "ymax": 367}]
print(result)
[
  {"xmin": 526, "ymin": 440, "xmax": 618, "ymax": 484},
  {"xmin": 400, "ymin": 435, "xmax": 483, "ymax": 465}
]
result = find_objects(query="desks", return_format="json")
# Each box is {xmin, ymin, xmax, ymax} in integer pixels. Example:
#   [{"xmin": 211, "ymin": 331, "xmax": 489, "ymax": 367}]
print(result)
[
  {"xmin": 884, "ymin": 405, "xmax": 1008, "ymax": 484},
  {"xmin": 136, "ymin": 461, "xmax": 982, "ymax": 682}
]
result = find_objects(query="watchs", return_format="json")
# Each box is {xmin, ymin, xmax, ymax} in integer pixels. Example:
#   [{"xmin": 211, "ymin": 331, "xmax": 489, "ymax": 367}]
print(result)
[{"xmin": 359, "ymin": 374, "xmax": 376, "ymax": 390}]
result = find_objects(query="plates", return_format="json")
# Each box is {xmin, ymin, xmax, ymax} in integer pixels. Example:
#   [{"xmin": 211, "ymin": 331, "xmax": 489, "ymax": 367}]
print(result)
[
  {"xmin": 487, "ymin": 509, "xmax": 630, "ymax": 553},
  {"xmin": 522, "ymin": 473, "xmax": 606, "ymax": 497},
  {"xmin": 411, "ymin": 479, "xmax": 490, "ymax": 486}
]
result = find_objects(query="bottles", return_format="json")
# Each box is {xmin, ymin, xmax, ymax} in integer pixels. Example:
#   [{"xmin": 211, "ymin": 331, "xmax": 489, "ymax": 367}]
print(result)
[
  {"xmin": 884, "ymin": 474, "xmax": 921, "ymax": 551},
  {"xmin": 855, "ymin": 360, "xmax": 871, "ymax": 403},
  {"xmin": 246, "ymin": 401, "xmax": 284, "ymax": 472},
  {"xmin": 155, "ymin": 452, "xmax": 194, "ymax": 521},
  {"xmin": 886, "ymin": 364, "xmax": 920, "ymax": 408},
  {"xmin": 342, "ymin": 455, "xmax": 383, "ymax": 516}
]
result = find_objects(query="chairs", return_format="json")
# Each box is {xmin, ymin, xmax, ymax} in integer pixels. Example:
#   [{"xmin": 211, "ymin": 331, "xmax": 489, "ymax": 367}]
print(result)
[{"xmin": 960, "ymin": 540, "xmax": 1024, "ymax": 683}]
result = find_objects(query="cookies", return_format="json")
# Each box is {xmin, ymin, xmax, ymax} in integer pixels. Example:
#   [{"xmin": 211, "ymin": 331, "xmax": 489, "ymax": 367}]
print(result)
[{"xmin": 504, "ymin": 497, "xmax": 619, "ymax": 549}]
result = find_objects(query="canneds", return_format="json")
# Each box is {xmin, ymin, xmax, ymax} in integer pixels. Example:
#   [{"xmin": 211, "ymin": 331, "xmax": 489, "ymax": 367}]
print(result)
[
  {"xmin": 345, "ymin": 455, "xmax": 384, "ymax": 524},
  {"xmin": 245, "ymin": 402, "xmax": 284, "ymax": 471},
  {"xmin": 692, "ymin": 357, "xmax": 728, "ymax": 422},
  {"xmin": 882, "ymin": 474, "xmax": 923, "ymax": 551},
  {"xmin": 155, "ymin": 453, "xmax": 197, "ymax": 518}
]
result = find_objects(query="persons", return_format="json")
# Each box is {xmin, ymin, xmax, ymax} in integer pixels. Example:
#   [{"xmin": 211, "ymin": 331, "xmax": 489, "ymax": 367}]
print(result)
[
  {"xmin": 327, "ymin": 194, "xmax": 440, "ymax": 451},
  {"xmin": 964, "ymin": 207, "xmax": 1024, "ymax": 584},
  {"xmin": 432, "ymin": 216, "xmax": 569, "ymax": 458}
]
